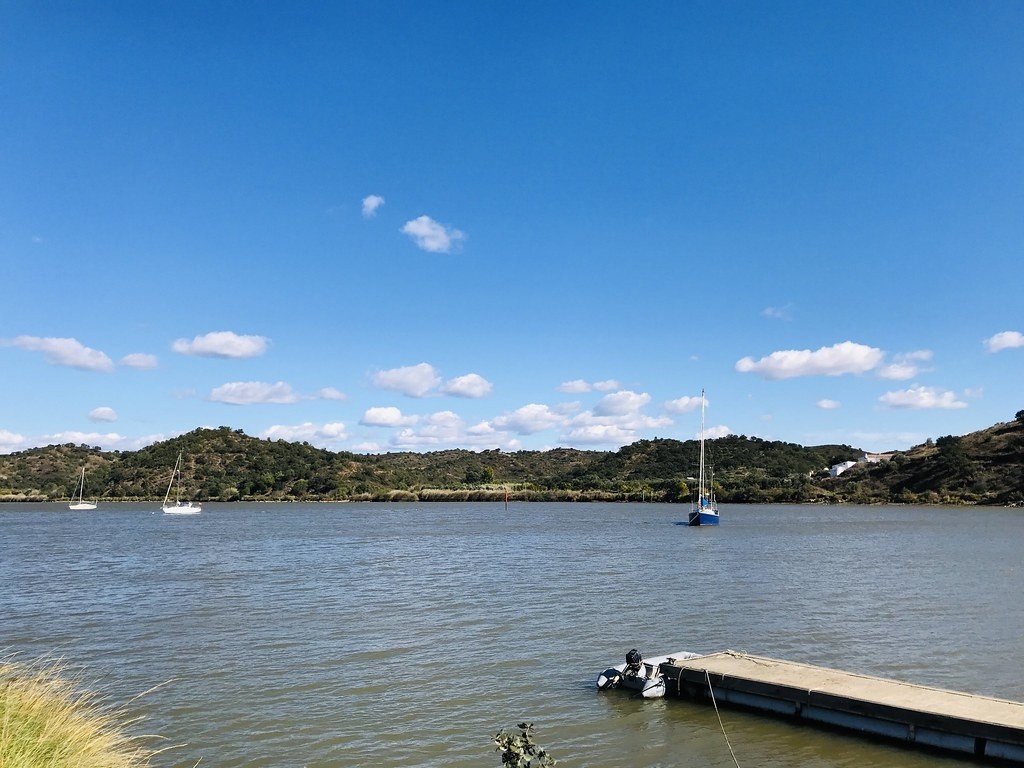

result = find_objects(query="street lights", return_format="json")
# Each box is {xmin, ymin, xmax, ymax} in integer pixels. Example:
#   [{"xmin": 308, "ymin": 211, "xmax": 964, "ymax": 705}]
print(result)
[{"xmin": 688, "ymin": 387, "xmax": 721, "ymax": 527}]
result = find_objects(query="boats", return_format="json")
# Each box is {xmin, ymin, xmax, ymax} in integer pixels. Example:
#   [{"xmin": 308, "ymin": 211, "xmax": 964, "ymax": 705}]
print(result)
[{"xmin": 596, "ymin": 647, "xmax": 705, "ymax": 699}]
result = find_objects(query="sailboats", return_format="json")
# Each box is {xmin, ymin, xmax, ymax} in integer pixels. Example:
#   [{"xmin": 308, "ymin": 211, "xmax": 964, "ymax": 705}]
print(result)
[
  {"xmin": 69, "ymin": 468, "xmax": 98, "ymax": 510},
  {"xmin": 161, "ymin": 452, "xmax": 203, "ymax": 516}
]
